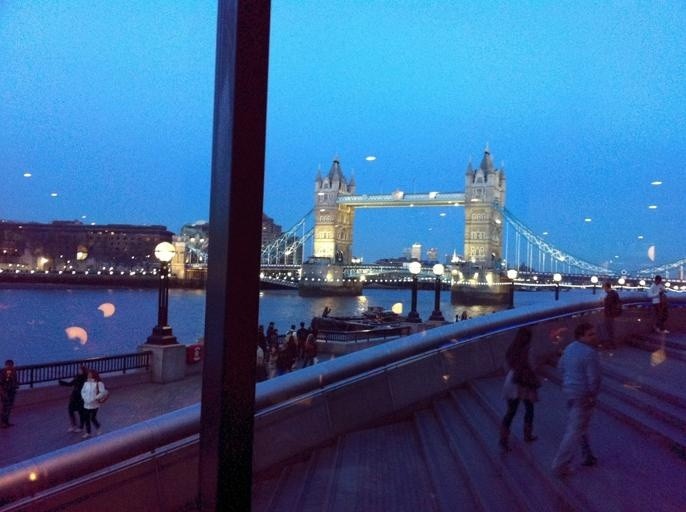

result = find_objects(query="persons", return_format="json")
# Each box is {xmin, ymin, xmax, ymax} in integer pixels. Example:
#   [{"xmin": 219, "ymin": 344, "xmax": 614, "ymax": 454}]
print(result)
[
  {"xmin": 80, "ymin": 369, "xmax": 109, "ymax": 440},
  {"xmin": 601, "ymin": 283, "xmax": 621, "ymax": 350},
  {"xmin": 495, "ymin": 324, "xmax": 543, "ymax": 451},
  {"xmin": 549, "ymin": 320, "xmax": 601, "ymax": 480},
  {"xmin": 256, "ymin": 320, "xmax": 317, "ymax": 382},
  {"xmin": 56, "ymin": 366, "xmax": 87, "ymax": 434},
  {"xmin": 646, "ymin": 274, "xmax": 671, "ymax": 335},
  {"xmin": 320, "ymin": 306, "xmax": 331, "ymax": 318},
  {"xmin": 0, "ymin": 359, "xmax": 16, "ymax": 428}
]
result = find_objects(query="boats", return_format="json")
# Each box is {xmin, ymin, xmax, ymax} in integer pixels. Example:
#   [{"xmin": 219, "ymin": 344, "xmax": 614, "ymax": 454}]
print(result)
[{"xmin": 312, "ymin": 305, "xmax": 407, "ymax": 334}]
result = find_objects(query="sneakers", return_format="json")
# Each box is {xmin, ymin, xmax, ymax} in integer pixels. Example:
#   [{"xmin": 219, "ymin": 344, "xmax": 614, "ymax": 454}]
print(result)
[
  {"xmin": 67, "ymin": 425, "xmax": 104, "ymax": 441},
  {"xmin": 524, "ymin": 433, "xmax": 538, "ymax": 442},
  {"xmin": 582, "ymin": 456, "xmax": 598, "ymax": 467},
  {"xmin": 498, "ymin": 436, "xmax": 512, "ymax": 451},
  {"xmin": 553, "ymin": 467, "xmax": 569, "ymax": 478},
  {"xmin": 0, "ymin": 422, "xmax": 15, "ymax": 429}
]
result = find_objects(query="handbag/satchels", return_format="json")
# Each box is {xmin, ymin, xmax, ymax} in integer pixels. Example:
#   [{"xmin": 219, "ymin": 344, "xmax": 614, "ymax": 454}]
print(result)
[
  {"xmin": 96, "ymin": 381, "xmax": 110, "ymax": 403},
  {"xmin": 511, "ymin": 365, "xmax": 542, "ymax": 389}
]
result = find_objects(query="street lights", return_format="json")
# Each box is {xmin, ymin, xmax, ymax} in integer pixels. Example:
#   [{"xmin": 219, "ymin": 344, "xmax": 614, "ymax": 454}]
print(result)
[
  {"xmin": 429, "ymin": 264, "xmax": 448, "ymax": 320},
  {"xmin": 591, "ymin": 275, "xmax": 598, "ymax": 295},
  {"xmin": 639, "ymin": 280, "xmax": 646, "ymax": 291},
  {"xmin": 149, "ymin": 241, "xmax": 179, "ymax": 345},
  {"xmin": 553, "ymin": 272, "xmax": 561, "ymax": 300},
  {"xmin": 406, "ymin": 260, "xmax": 423, "ymax": 322},
  {"xmin": 665, "ymin": 281, "xmax": 686, "ymax": 293},
  {"xmin": 507, "ymin": 269, "xmax": 519, "ymax": 309},
  {"xmin": 618, "ymin": 277, "xmax": 626, "ymax": 292}
]
